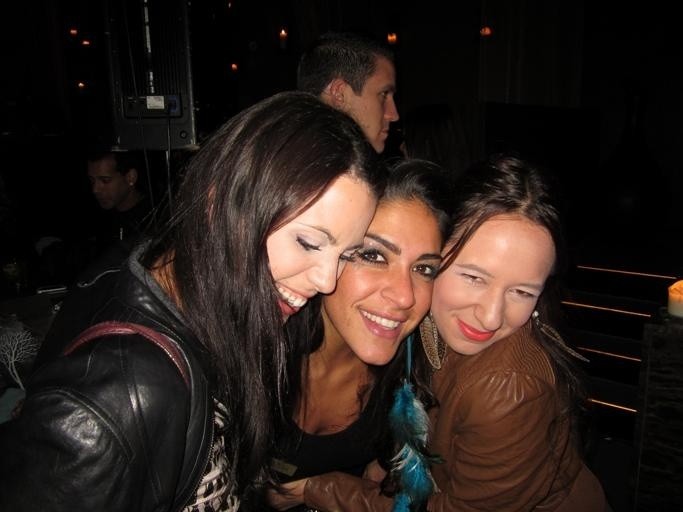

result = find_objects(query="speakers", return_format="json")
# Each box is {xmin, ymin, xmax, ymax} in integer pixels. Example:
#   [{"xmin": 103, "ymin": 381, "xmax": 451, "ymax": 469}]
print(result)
[{"xmin": 105, "ymin": 0, "xmax": 198, "ymax": 150}]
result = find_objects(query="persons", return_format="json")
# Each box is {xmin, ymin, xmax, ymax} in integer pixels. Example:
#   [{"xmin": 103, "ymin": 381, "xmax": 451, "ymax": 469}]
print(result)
[
  {"xmin": 266, "ymin": 153, "xmax": 607, "ymax": 512},
  {"xmin": 0, "ymin": 89, "xmax": 387, "ymax": 512},
  {"xmin": 293, "ymin": 30, "xmax": 399, "ymax": 156},
  {"xmin": 236, "ymin": 157, "xmax": 464, "ymax": 512},
  {"xmin": 9, "ymin": 136, "xmax": 155, "ymax": 419}
]
